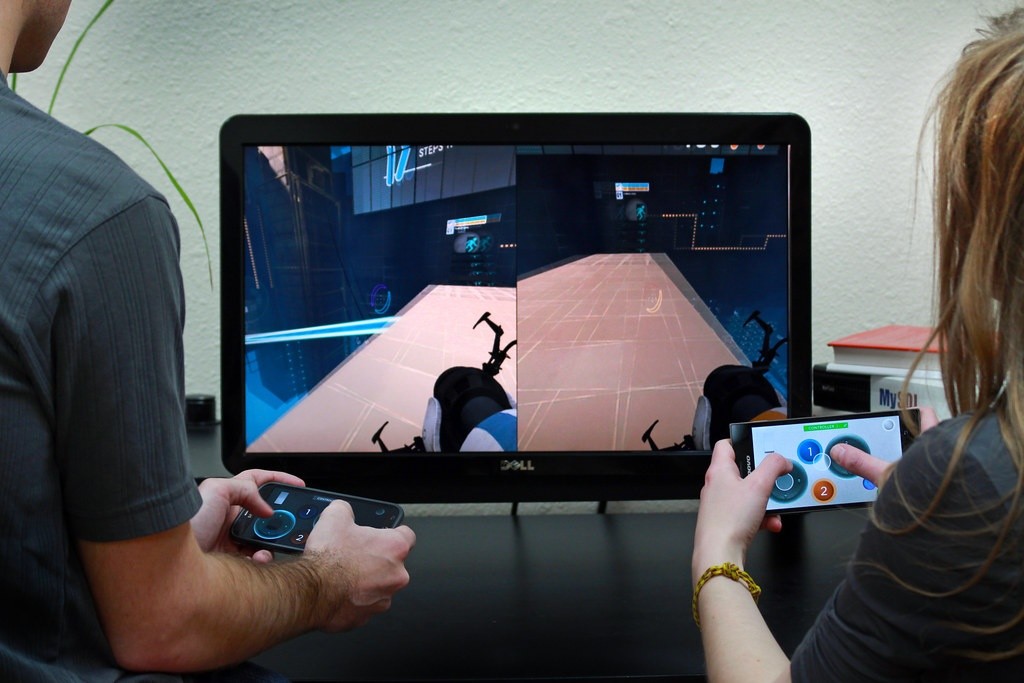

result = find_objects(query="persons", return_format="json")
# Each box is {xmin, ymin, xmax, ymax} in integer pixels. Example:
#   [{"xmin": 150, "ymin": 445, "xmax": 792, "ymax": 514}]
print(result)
[
  {"xmin": 690, "ymin": 0, "xmax": 1024, "ymax": 683},
  {"xmin": 0, "ymin": 0, "xmax": 414, "ymax": 683}
]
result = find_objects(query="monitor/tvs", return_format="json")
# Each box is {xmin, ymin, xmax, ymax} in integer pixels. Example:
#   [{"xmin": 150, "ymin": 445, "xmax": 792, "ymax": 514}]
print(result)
[{"xmin": 218, "ymin": 112, "xmax": 812, "ymax": 507}]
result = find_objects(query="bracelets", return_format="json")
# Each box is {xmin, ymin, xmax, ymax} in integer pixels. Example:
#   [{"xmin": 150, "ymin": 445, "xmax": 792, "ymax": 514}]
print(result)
[{"xmin": 693, "ymin": 563, "xmax": 761, "ymax": 626}]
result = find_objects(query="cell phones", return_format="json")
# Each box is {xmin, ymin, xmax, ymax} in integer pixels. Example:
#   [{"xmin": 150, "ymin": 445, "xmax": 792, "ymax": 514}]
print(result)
[
  {"xmin": 730, "ymin": 408, "xmax": 922, "ymax": 515},
  {"xmin": 227, "ymin": 481, "xmax": 405, "ymax": 554}
]
